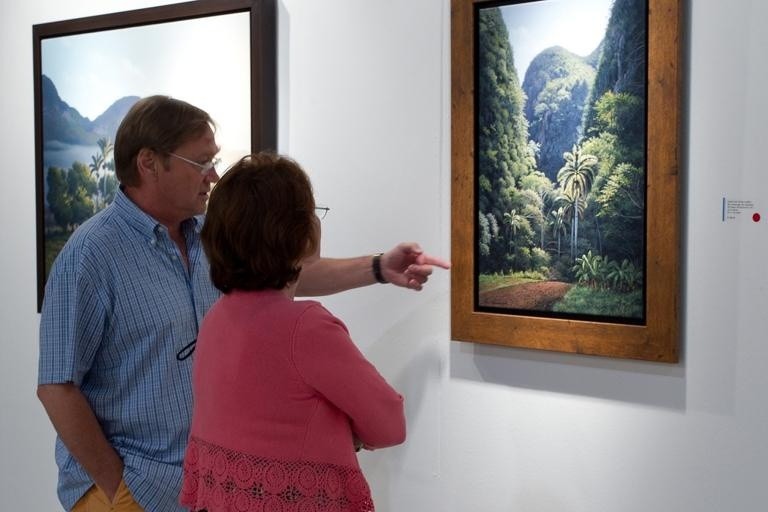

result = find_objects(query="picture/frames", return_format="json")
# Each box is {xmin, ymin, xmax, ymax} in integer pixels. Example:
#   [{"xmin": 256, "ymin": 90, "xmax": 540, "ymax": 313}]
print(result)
[
  {"xmin": 450, "ymin": 0, "xmax": 680, "ymax": 364},
  {"xmin": 32, "ymin": 0, "xmax": 276, "ymax": 314}
]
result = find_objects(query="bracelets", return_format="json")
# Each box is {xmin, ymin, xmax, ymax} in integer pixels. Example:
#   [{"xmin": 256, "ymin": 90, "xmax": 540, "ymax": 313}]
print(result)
[{"xmin": 371, "ymin": 249, "xmax": 389, "ymax": 286}]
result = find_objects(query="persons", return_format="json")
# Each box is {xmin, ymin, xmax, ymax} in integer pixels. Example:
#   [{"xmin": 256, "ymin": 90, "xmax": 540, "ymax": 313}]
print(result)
[
  {"xmin": 175, "ymin": 150, "xmax": 411, "ymax": 511},
  {"xmin": 36, "ymin": 96, "xmax": 452, "ymax": 512}
]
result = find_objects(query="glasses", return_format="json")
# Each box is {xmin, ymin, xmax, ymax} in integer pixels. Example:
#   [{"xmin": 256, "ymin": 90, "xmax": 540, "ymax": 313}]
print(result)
[
  {"xmin": 168, "ymin": 151, "xmax": 222, "ymax": 175},
  {"xmin": 311, "ymin": 206, "xmax": 330, "ymax": 222}
]
